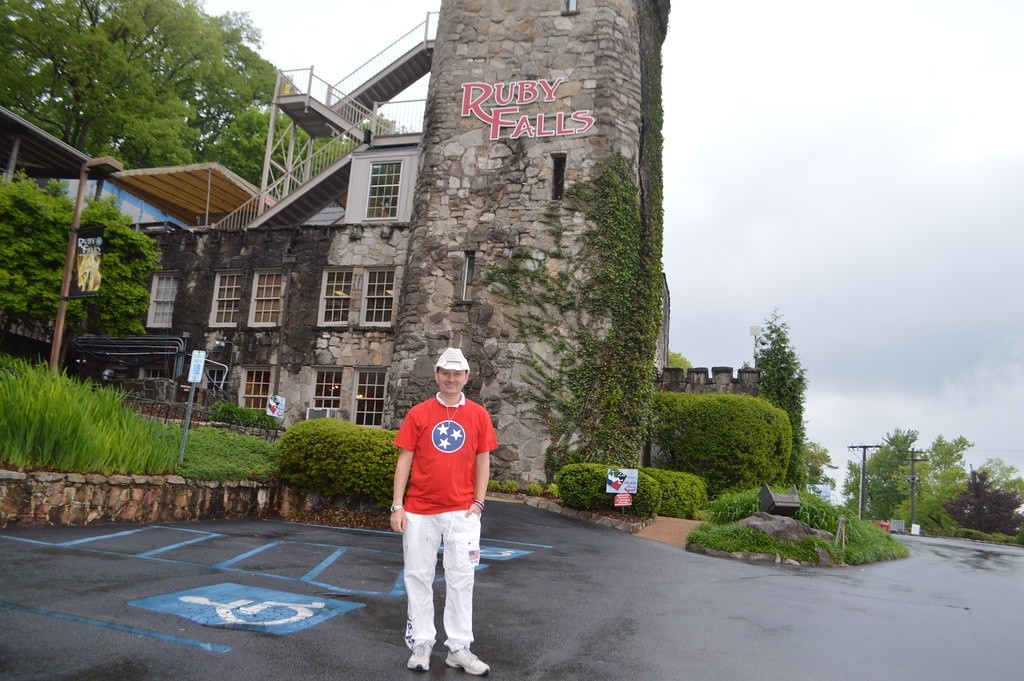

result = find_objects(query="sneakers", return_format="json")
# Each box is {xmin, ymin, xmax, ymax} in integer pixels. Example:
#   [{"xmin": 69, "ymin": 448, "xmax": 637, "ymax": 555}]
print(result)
[
  {"xmin": 445, "ymin": 648, "xmax": 490, "ymax": 675},
  {"xmin": 407, "ymin": 642, "xmax": 433, "ymax": 671}
]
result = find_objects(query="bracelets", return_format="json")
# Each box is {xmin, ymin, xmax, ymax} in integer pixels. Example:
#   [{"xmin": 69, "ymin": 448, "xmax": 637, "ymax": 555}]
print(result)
[{"xmin": 474, "ymin": 497, "xmax": 485, "ymax": 510}]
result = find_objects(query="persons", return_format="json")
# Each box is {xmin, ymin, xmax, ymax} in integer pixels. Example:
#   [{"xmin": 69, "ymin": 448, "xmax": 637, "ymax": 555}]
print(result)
[{"xmin": 389, "ymin": 347, "xmax": 499, "ymax": 677}]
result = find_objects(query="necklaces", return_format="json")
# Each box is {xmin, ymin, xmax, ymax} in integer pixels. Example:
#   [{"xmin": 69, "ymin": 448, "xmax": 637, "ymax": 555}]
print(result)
[{"xmin": 439, "ymin": 393, "xmax": 462, "ymax": 429}]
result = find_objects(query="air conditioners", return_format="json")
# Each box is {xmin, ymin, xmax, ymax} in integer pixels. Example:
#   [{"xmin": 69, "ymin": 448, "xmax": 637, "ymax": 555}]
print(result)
[{"xmin": 304, "ymin": 407, "xmax": 338, "ymax": 422}]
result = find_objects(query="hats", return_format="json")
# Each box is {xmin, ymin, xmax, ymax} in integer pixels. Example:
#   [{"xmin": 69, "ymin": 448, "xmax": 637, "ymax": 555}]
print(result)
[{"xmin": 434, "ymin": 347, "xmax": 471, "ymax": 375}]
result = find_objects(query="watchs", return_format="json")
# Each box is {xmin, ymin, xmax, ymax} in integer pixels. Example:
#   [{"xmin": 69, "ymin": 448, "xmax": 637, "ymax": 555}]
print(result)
[{"xmin": 390, "ymin": 504, "xmax": 404, "ymax": 513}]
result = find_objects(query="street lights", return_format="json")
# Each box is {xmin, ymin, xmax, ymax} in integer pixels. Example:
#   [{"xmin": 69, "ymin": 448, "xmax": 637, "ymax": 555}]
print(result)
[{"xmin": 50, "ymin": 152, "xmax": 124, "ymax": 374}]
